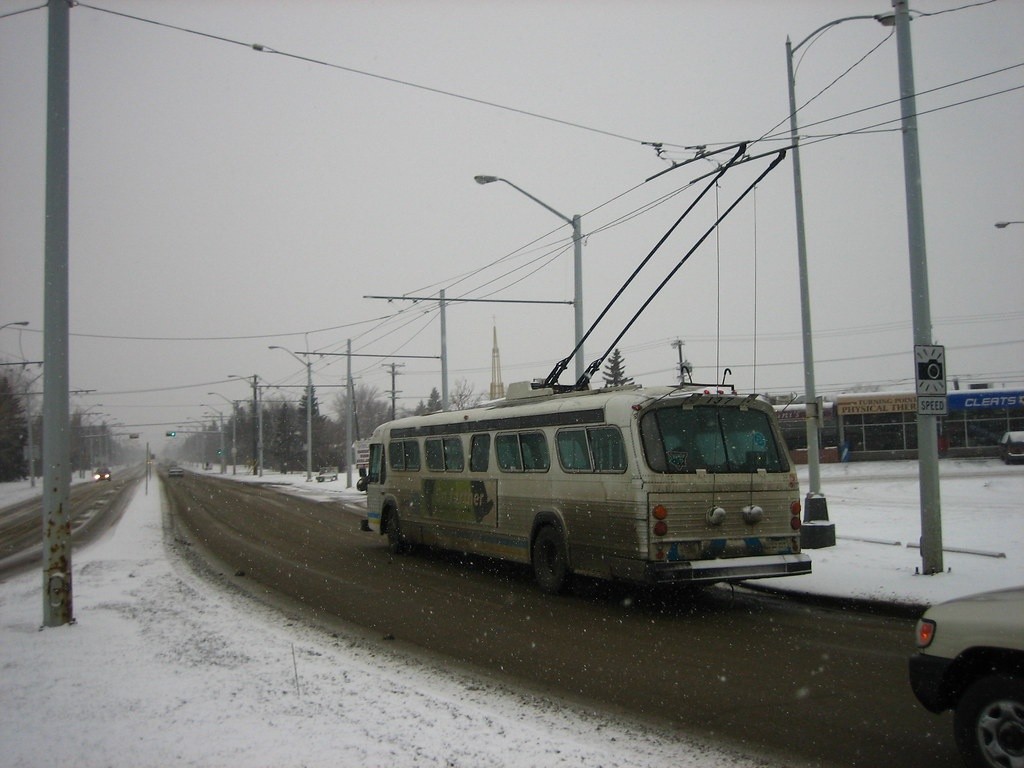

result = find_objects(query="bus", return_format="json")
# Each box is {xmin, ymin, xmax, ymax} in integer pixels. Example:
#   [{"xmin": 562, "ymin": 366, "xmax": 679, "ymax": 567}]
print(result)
[{"xmin": 355, "ymin": 380, "xmax": 815, "ymax": 601}]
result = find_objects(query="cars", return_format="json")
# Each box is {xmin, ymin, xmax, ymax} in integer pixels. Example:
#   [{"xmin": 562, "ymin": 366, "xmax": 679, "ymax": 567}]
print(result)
[
  {"xmin": 94, "ymin": 468, "xmax": 112, "ymax": 482},
  {"xmin": 168, "ymin": 467, "xmax": 184, "ymax": 477},
  {"xmin": 997, "ymin": 431, "xmax": 1024, "ymax": 465},
  {"xmin": 910, "ymin": 584, "xmax": 1024, "ymax": 768}
]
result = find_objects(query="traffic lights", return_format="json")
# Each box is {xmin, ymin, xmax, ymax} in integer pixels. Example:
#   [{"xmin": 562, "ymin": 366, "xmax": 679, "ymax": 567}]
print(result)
[{"xmin": 166, "ymin": 432, "xmax": 176, "ymax": 436}]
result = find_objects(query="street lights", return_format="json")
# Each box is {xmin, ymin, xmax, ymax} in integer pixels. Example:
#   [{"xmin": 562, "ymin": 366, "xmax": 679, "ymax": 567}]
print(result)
[
  {"xmin": 268, "ymin": 343, "xmax": 314, "ymax": 482},
  {"xmin": 783, "ymin": 11, "xmax": 915, "ymax": 548},
  {"xmin": 180, "ymin": 375, "xmax": 264, "ymax": 477},
  {"xmin": 0, "ymin": 320, "xmax": 125, "ymax": 488},
  {"xmin": 473, "ymin": 173, "xmax": 585, "ymax": 391}
]
